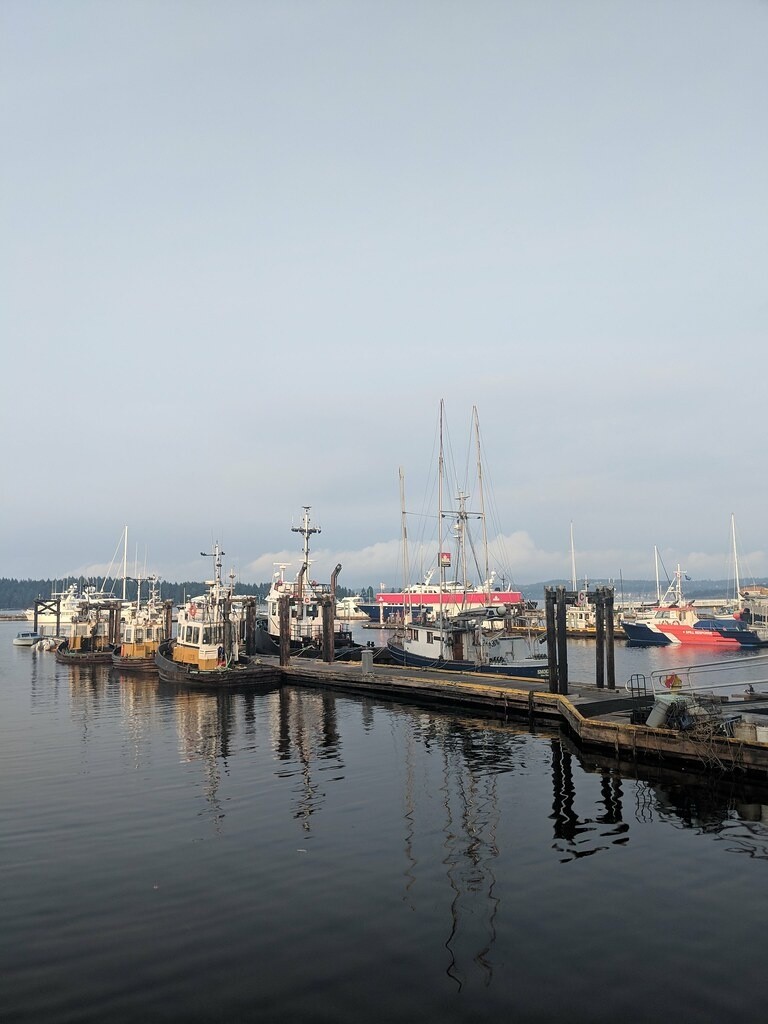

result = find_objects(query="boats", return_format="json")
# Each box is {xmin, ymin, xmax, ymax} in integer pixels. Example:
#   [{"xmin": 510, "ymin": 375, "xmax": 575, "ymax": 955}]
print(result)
[{"xmin": 12, "ymin": 398, "xmax": 768, "ymax": 695}]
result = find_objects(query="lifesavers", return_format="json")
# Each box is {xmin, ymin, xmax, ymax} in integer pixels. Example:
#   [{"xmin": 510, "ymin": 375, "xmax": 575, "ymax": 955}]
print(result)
[
  {"xmin": 189, "ymin": 605, "xmax": 197, "ymax": 615},
  {"xmin": 663, "ymin": 620, "xmax": 670, "ymax": 624},
  {"xmin": 672, "ymin": 621, "xmax": 680, "ymax": 626}
]
